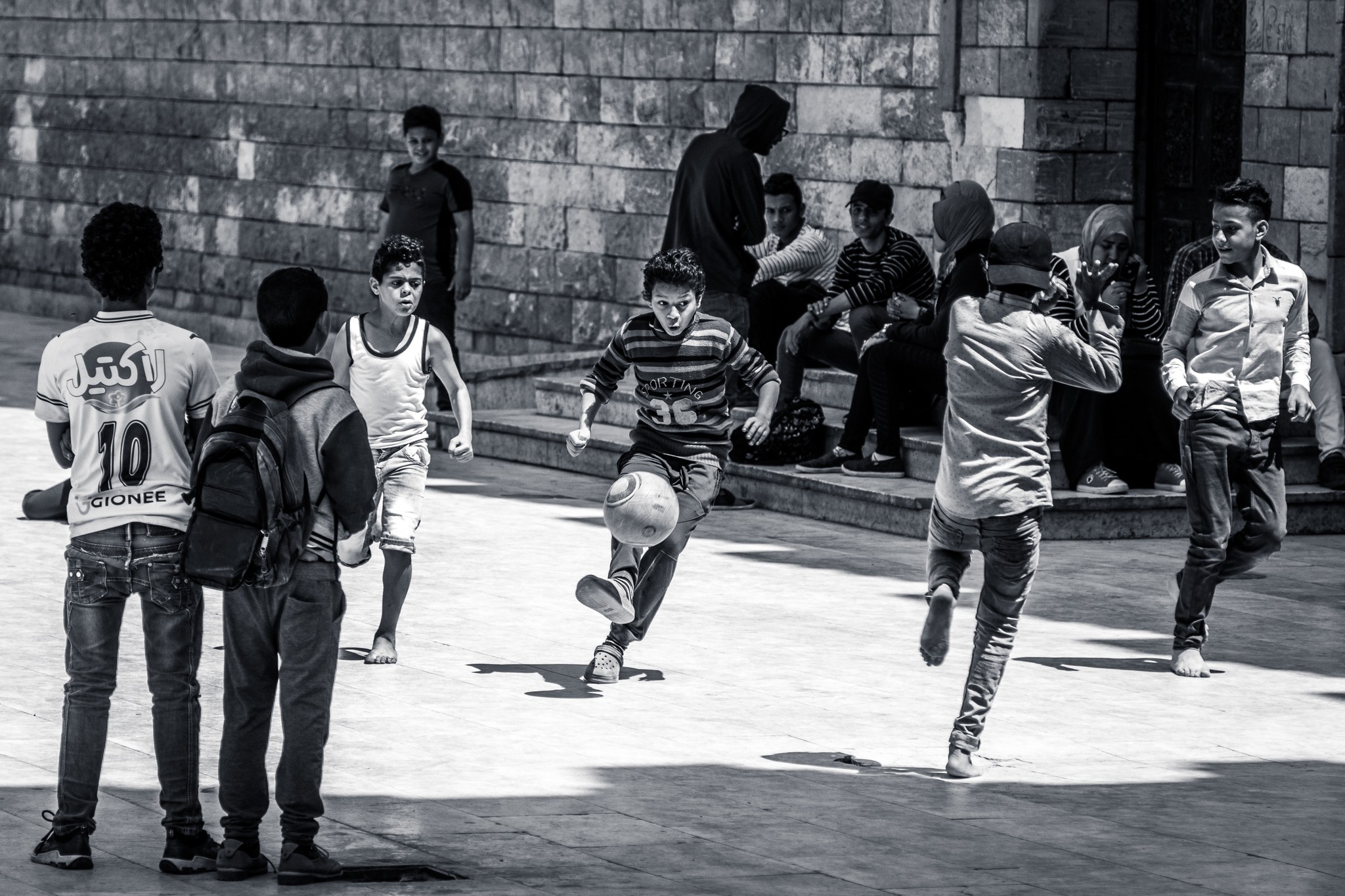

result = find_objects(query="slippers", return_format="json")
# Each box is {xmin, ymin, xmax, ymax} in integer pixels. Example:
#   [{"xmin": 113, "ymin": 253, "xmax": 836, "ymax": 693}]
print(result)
[
  {"xmin": 584, "ymin": 645, "xmax": 624, "ymax": 684},
  {"xmin": 576, "ymin": 574, "xmax": 635, "ymax": 624}
]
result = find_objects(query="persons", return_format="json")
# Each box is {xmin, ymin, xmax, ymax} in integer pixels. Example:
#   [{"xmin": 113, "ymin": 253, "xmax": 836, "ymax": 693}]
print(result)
[
  {"xmin": 775, "ymin": 180, "xmax": 941, "ymax": 430},
  {"xmin": 565, "ymin": 247, "xmax": 783, "ymax": 686},
  {"xmin": 734, "ymin": 171, "xmax": 856, "ymax": 410},
  {"xmin": 186, "ymin": 265, "xmax": 376, "ymax": 882},
  {"xmin": 1161, "ymin": 175, "xmax": 1318, "ymax": 677},
  {"xmin": 1037, "ymin": 205, "xmax": 1188, "ymax": 494},
  {"xmin": 331, "ymin": 234, "xmax": 473, "ymax": 664},
  {"xmin": 658, "ymin": 84, "xmax": 792, "ymax": 512},
  {"xmin": 33, "ymin": 201, "xmax": 221, "ymax": 875},
  {"xmin": 794, "ymin": 180, "xmax": 997, "ymax": 478},
  {"xmin": 377, "ymin": 104, "xmax": 474, "ymax": 412},
  {"xmin": 918, "ymin": 221, "xmax": 1126, "ymax": 778},
  {"xmin": 1164, "ymin": 232, "xmax": 1345, "ymax": 492}
]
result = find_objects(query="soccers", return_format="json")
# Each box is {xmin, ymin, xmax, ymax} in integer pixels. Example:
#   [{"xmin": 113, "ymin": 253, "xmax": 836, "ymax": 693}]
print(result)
[{"xmin": 603, "ymin": 470, "xmax": 681, "ymax": 548}]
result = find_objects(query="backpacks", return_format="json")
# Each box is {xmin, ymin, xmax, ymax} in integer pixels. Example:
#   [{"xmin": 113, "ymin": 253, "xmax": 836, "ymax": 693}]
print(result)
[{"xmin": 180, "ymin": 382, "xmax": 345, "ymax": 592}]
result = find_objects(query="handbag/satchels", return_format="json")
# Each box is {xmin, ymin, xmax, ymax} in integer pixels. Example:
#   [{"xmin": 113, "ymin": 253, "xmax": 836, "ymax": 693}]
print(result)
[{"xmin": 729, "ymin": 398, "xmax": 826, "ymax": 466}]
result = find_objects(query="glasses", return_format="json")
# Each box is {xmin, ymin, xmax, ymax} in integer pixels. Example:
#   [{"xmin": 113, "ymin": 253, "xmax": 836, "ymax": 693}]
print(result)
[{"xmin": 768, "ymin": 127, "xmax": 788, "ymax": 137}]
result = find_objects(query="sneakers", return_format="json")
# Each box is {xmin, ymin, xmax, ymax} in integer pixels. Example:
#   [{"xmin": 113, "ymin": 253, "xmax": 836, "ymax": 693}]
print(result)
[
  {"xmin": 30, "ymin": 810, "xmax": 93, "ymax": 871},
  {"xmin": 1076, "ymin": 463, "xmax": 1128, "ymax": 494},
  {"xmin": 795, "ymin": 450, "xmax": 842, "ymax": 474},
  {"xmin": 841, "ymin": 453, "xmax": 906, "ymax": 478},
  {"xmin": 215, "ymin": 831, "xmax": 276, "ymax": 880},
  {"xmin": 710, "ymin": 489, "xmax": 756, "ymax": 510},
  {"xmin": 159, "ymin": 828, "xmax": 221, "ymax": 876},
  {"xmin": 1155, "ymin": 463, "xmax": 1186, "ymax": 493},
  {"xmin": 277, "ymin": 837, "xmax": 343, "ymax": 884}
]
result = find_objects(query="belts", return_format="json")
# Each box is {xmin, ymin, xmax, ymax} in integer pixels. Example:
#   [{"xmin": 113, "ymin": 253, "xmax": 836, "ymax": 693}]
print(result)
[{"xmin": 99, "ymin": 521, "xmax": 181, "ymax": 534}]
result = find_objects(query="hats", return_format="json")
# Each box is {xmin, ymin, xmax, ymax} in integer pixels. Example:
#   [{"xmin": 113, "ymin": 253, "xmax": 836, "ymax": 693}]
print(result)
[
  {"xmin": 988, "ymin": 221, "xmax": 1052, "ymax": 290},
  {"xmin": 846, "ymin": 180, "xmax": 894, "ymax": 213}
]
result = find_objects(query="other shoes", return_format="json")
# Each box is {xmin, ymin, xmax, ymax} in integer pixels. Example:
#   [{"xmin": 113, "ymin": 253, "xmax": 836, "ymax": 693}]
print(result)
[{"xmin": 1316, "ymin": 454, "xmax": 1345, "ymax": 490}]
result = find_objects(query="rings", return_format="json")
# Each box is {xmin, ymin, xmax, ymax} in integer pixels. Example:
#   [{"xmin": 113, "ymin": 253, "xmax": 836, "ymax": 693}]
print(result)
[
  {"xmin": 1121, "ymin": 292, "xmax": 1124, "ymax": 297},
  {"xmin": 894, "ymin": 296, "xmax": 899, "ymax": 300}
]
